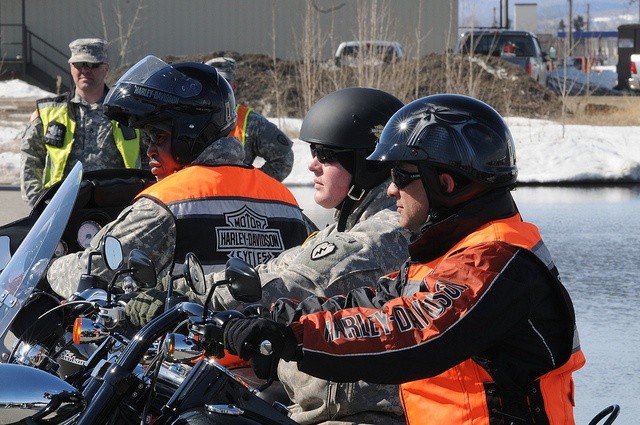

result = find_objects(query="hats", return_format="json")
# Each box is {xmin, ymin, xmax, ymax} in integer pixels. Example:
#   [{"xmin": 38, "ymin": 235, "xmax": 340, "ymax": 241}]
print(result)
[
  {"xmin": 66, "ymin": 37, "xmax": 110, "ymax": 64},
  {"xmin": 203, "ymin": 58, "xmax": 236, "ymax": 83}
]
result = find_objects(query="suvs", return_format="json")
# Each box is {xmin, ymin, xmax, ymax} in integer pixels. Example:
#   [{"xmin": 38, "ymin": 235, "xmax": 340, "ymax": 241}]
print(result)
[
  {"xmin": 455, "ymin": 30, "xmax": 547, "ymax": 92},
  {"xmin": 559, "ymin": 57, "xmax": 619, "ymax": 92}
]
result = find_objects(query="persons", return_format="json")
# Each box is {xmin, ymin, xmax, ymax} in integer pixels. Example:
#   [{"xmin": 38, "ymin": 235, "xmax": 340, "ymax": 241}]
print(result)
[
  {"xmin": 46, "ymin": 55, "xmax": 317, "ymax": 298},
  {"xmin": 189, "ymin": 57, "xmax": 294, "ymax": 182},
  {"xmin": 19, "ymin": 39, "xmax": 150, "ymax": 205},
  {"xmin": 126, "ymin": 88, "xmax": 411, "ymax": 425},
  {"xmin": 221, "ymin": 95, "xmax": 586, "ymax": 425}
]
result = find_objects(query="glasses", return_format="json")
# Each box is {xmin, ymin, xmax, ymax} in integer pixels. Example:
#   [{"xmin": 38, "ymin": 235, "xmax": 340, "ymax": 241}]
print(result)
[
  {"xmin": 309, "ymin": 145, "xmax": 340, "ymax": 163},
  {"xmin": 73, "ymin": 62, "xmax": 99, "ymax": 69},
  {"xmin": 391, "ymin": 168, "xmax": 420, "ymax": 188},
  {"xmin": 140, "ymin": 129, "xmax": 167, "ymax": 145}
]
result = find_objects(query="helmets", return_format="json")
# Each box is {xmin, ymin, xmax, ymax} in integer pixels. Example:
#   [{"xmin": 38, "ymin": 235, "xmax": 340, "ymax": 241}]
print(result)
[
  {"xmin": 102, "ymin": 55, "xmax": 236, "ymax": 157},
  {"xmin": 365, "ymin": 94, "xmax": 516, "ymax": 193},
  {"xmin": 300, "ymin": 89, "xmax": 405, "ymax": 187}
]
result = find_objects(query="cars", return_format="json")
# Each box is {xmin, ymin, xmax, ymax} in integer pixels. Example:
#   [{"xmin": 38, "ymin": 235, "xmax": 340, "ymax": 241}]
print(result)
[
  {"xmin": 329, "ymin": 42, "xmax": 405, "ymax": 86},
  {"xmin": 203, "ymin": 58, "xmax": 237, "ymax": 95},
  {"xmin": 629, "ymin": 54, "xmax": 640, "ymax": 90}
]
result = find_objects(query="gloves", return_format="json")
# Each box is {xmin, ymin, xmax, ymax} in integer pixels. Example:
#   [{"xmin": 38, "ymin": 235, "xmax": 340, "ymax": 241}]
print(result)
[
  {"xmin": 128, "ymin": 290, "xmax": 189, "ymax": 328},
  {"xmin": 224, "ymin": 317, "xmax": 296, "ymax": 361}
]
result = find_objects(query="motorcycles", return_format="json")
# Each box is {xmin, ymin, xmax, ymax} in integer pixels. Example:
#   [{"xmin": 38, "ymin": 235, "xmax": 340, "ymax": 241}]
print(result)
[{"xmin": 1, "ymin": 258, "xmax": 619, "ymax": 425}]
